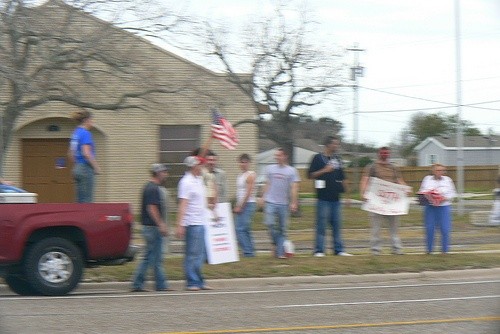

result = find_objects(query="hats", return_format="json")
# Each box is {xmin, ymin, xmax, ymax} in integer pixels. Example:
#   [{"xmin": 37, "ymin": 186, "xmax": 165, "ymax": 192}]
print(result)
[
  {"xmin": 184, "ymin": 156, "xmax": 200, "ymax": 167},
  {"xmin": 150, "ymin": 164, "xmax": 167, "ymax": 172}
]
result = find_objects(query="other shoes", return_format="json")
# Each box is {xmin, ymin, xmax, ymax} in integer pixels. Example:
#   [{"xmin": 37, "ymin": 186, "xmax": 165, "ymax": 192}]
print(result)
[
  {"xmin": 336, "ymin": 252, "xmax": 353, "ymax": 256},
  {"xmin": 277, "ymin": 256, "xmax": 287, "ymax": 259},
  {"xmin": 186, "ymin": 287, "xmax": 200, "ymax": 291},
  {"xmin": 313, "ymin": 252, "xmax": 325, "ymax": 257},
  {"xmin": 156, "ymin": 288, "xmax": 174, "ymax": 291},
  {"xmin": 200, "ymin": 286, "xmax": 212, "ymax": 290},
  {"xmin": 130, "ymin": 287, "xmax": 151, "ymax": 292}
]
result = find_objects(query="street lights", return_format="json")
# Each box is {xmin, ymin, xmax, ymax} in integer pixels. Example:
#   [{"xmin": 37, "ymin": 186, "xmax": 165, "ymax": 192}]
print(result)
[{"xmin": 347, "ymin": 42, "xmax": 366, "ymax": 193}]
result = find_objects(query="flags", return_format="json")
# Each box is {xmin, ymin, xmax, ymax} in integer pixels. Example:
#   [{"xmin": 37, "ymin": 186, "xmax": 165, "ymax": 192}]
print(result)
[{"xmin": 210, "ymin": 106, "xmax": 241, "ymax": 152}]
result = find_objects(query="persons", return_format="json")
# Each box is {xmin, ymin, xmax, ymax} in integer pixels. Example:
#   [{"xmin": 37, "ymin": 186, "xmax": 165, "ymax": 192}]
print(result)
[
  {"xmin": 190, "ymin": 133, "xmax": 226, "ymax": 211},
  {"xmin": 418, "ymin": 162, "xmax": 459, "ymax": 256},
  {"xmin": 175, "ymin": 155, "xmax": 213, "ymax": 291},
  {"xmin": 359, "ymin": 146, "xmax": 413, "ymax": 255},
  {"xmin": 233, "ymin": 152, "xmax": 259, "ymax": 257},
  {"xmin": 305, "ymin": 134, "xmax": 353, "ymax": 257},
  {"xmin": 259, "ymin": 147, "xmax": 301, "ymax": 259},
  {"xmin": 67, "ymin": 109, "xmax": 103, "ymax": 203},
  {"xmin": 127, "ymin": 163, "xmax": 176, "ymax": 292}
]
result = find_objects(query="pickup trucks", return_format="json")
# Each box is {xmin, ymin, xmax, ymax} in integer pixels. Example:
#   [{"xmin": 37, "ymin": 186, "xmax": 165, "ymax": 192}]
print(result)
[{"xmin": 0, "ymin": 201, "xmax": 139, "ymax": 297}]
